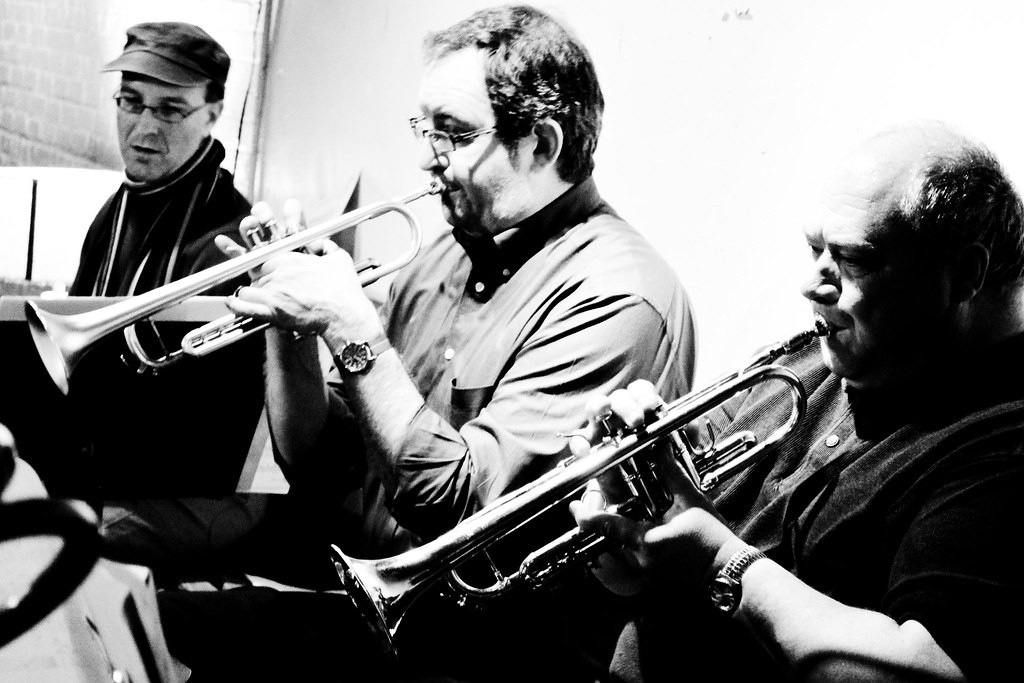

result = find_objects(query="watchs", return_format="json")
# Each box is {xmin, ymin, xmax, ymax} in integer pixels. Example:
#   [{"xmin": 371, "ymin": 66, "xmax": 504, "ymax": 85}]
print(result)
[
  {"xmin": 706, "ymin": 545, "xmax": 767, "ymax": 621},
  {"xmin": 327, "ymin": 330, "xmax": 395, "ymax": 379}
]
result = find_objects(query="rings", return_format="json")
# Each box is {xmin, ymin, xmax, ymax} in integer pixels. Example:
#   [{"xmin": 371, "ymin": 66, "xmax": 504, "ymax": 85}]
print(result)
[{"xmin": 232, "ymin": 283, "xmax": 244, "ymax": 297}]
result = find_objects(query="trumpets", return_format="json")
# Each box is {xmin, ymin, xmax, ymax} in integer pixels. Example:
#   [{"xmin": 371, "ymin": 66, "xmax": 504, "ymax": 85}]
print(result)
[
  {"xmin": 329, "ymin": 312, "xmax": 829, "ymax": 667},
  {"xmin": 22, "ymin": 181, "xmax": 446, "ymax": 399}
]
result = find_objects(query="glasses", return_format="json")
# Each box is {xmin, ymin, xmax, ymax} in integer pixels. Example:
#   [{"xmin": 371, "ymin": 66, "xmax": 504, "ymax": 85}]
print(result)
[
  {"xmin": 114, "ymin": 92, "xmax": 210, "ymax": 125},
  {"xmin": 409, "ymin": 114, "xmax": 495, "ymax": 152}
]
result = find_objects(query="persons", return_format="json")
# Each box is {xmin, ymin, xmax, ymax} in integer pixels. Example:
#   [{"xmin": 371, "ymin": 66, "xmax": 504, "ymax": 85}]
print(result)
[
  {"xmin": 0, "ymin": 14, "xmax": 268, "ymax": 545},
  {"xmin": 149, "ymin": 3, "xmax": 700, "ymax": 683},
  {"xmin": 566, "ymin": 115, "xmax": 1023, "ymax": 683}
]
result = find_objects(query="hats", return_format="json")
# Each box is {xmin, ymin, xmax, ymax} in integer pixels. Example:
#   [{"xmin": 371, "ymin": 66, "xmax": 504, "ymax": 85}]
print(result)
[{"xmin": 98, "ymin": 22, "xmax": 230, "ymax": 87}]
